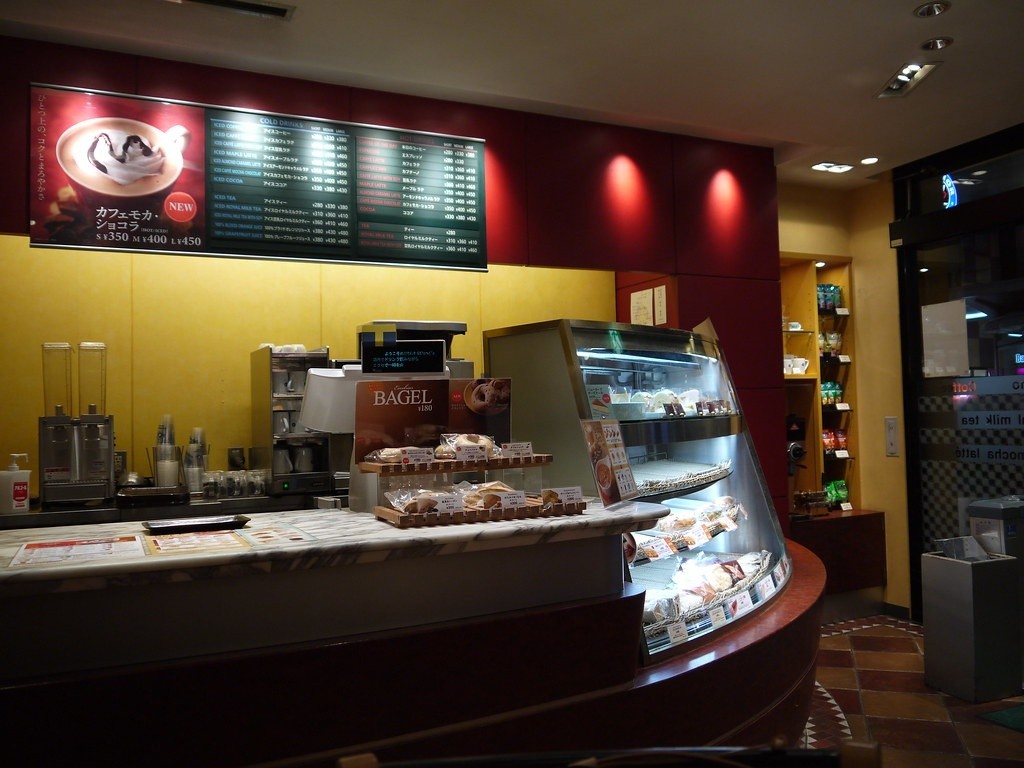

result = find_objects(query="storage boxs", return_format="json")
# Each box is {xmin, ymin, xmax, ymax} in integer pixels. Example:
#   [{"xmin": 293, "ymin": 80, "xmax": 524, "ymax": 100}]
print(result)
[{"xmin": 923, "ymin": 548, "xmax": 1019, "ymax": 702}]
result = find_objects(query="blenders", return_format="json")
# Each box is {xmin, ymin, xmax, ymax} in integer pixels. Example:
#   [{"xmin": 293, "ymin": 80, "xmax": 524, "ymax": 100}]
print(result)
[{"xmin": 36, "ymin": 340, "xmax": 116, "ymax": 507}]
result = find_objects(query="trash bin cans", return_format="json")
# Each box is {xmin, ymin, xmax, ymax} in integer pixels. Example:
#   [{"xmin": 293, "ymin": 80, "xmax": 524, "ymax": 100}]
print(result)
[{"xmin": 923, "ymin": 536, "xmax": 1023, "ymax": 703}]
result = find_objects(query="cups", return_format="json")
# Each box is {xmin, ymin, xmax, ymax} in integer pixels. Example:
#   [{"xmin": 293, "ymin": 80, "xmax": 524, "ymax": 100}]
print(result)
[
  {"xmin": 248, "ymin": 468, "xmax": 270, "ymax": 497},
  {"xmin": 201, "ymin": 468, "xmax": 228, "ymax": 501},
  {"xmin": 273, "ymin": 369, "xmax": 313, "ymax": 474},
  {"xmin": 787, "ymin": 321, "xmax": 802, "ymax": 330},
  {"xmin": 56, "ymin": 116, "xmax": 190, "ymax": 227},
  {"xmin": 154, "ymin": 412, "xmax": 210, "ymax": 493},
  {"xmin": 792, "ymin": 357, "xmax": 810, "ymax": 375},
  {"xmin": 784, "ymin": 358, "xmax": 793, "ymax": 376},
  {"xmin": 223, "ymin": 469, "xmax": 250, "ymax": 498}
]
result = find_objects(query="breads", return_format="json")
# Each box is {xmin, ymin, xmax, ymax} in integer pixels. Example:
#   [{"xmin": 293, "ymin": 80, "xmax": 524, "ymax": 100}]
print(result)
[
  {"xmin": 374, "ymin": 434, "xmax": 518, "ymax": 513},
  {"xmin": 637, "ymin": 496, "xmax": 770, "ymax": 621},
  {"xmin": 612, "ymin": 388, "xmax": 722, "ymax": 414}
]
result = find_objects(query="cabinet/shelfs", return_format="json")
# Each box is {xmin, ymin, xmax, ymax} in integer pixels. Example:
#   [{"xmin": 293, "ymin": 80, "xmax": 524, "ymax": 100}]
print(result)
[
  {"xmin": 779, "ymin": 250, "xmax": 858, "ymax": 507},
  {"xmin": 359, "ymin": 452, "xmax": 587, "ymax": 529},
  {"xmin": 251, "ymin": 345, "xmax": 333, "ymax": 498},
  {"xmin": 791, "ymin": 509, "xmax": 887, "ymax": 623},
  {"xmin": 481, "ymin": 320, "xmax": 793, "ymax": 672},
  {"xmin": 329, "ymin": 360, "xmax": 475, "ymax": 493}
]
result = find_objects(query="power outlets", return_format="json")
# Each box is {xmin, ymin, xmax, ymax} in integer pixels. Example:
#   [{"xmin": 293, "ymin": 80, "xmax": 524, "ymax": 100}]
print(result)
[
  {"xmin": 228, "ymin": 448, "xmax": 243, "ymax": 469},
  {"xmin": 885, "ymin": 417, "xmax": 899, "ymax": 457}
]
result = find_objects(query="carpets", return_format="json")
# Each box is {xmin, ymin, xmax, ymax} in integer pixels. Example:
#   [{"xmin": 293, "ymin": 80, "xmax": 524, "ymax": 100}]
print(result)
[{"xmin": 797, "ymin": 682, "xmax": 851, "ymax": 750}]
